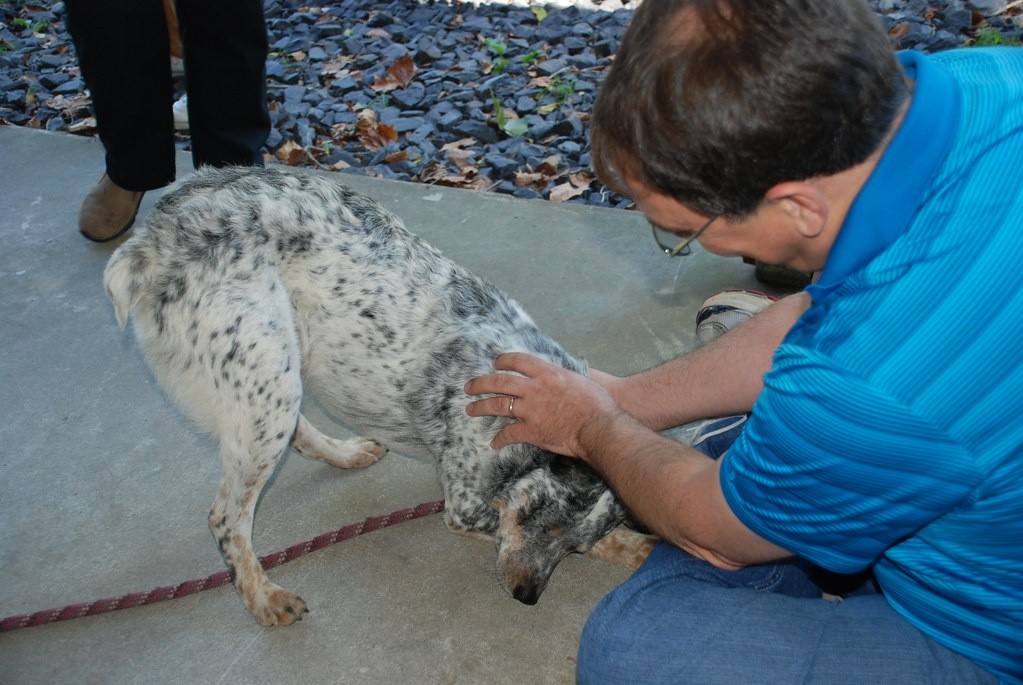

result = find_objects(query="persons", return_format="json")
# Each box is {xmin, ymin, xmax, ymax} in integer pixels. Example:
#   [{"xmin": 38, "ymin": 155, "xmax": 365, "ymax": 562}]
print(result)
[
  {"xmin": 463, "ymin": 0, "xmax": 1023, "ymax": 685},
  {"xmin": 63, "ymin": 0, "xmax": 268, "ymax": 243}
]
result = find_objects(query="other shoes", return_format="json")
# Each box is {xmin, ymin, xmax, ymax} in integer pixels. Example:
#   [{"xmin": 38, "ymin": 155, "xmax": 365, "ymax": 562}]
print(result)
[{"xmin": 696, "ymin": 288, "xmax": 779, "ymax": 346}]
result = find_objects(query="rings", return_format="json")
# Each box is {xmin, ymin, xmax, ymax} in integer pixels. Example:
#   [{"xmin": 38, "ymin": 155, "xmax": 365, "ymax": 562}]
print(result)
[{"xmin": 508, "ymin": 398, "xmax": 514, "ymax": 418}]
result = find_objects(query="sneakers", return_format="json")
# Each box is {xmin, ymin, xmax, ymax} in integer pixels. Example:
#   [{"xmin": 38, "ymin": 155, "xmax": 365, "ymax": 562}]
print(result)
[{"xmin": 79, "ymin": 169, "xmax": 146, "ymax": 243}]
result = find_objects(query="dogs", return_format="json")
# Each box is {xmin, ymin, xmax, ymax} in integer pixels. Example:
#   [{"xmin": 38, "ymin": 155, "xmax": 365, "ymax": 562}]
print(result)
[{"xmin": 106, "ymin": 164, "xmax": 624, "ymax": 628}]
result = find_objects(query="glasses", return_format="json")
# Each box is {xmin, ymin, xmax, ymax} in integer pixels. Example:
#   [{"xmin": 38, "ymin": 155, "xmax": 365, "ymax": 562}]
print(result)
[{"xmin": 652, "ymin": 214, "xmax": 719, "ymax": 257}]
